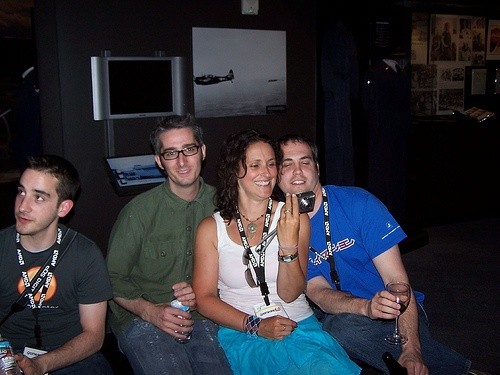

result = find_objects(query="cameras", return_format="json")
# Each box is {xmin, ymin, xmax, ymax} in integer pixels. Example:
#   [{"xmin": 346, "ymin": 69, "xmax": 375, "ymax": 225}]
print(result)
[{"xmin": 290, "ymin": 190, "xmax": 315, "ymax": 214}]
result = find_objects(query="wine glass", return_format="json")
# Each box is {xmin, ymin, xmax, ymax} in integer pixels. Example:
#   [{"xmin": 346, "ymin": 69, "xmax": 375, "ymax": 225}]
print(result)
[{"xmin": 385, "ymin": 281, "xmax": 411, "ymax": 345}]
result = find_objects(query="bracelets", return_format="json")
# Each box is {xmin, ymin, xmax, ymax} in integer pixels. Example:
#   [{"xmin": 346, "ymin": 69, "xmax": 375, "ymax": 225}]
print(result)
[
  {"xmin": 278, "ymin": 246, "xmax": 298, "ymax": 250},
  {"xmin": 242, "ymin": 314, "xmax": 249, "ymax": 331},
  {"xmin": 245, "ymin": 315, "xmax": 262, "ymax": 336},
  {"xmin": 368, "ymin": 300, "xmax": 377, "ymax": 319}
]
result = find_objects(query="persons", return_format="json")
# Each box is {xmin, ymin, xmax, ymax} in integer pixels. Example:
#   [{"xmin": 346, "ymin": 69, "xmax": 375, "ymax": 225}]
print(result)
[
  {"xmin": 191, "ymin": 128, "xmax": 363, "ymax": 375},
  {"xmin": 105, "ymin": 115, "xmax": 236, "ymax": 375},
  {"xmin": 0, "ymin": 156, "xmax": 113, "ymax": 375},
  {"xmin": 274, "ymin": 130, "xmax": 491, "ymax": 375}
]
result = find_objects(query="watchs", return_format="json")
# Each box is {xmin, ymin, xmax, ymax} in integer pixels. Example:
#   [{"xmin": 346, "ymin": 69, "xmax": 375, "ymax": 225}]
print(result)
[{"xmin": 278, "ymin": 251, "xmax": 298, "ymax": 262}]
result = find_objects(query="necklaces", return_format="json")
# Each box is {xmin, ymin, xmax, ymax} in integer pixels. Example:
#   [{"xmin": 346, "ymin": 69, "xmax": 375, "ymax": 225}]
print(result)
[{"xmin": 239, "ymin": 211, "xmax": 266, "ymax": 233}]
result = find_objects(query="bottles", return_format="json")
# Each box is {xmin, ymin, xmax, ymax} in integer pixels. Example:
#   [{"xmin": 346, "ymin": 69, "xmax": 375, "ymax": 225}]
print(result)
[
  {"xmin": 0, "ymin": 334, "xmax": 21, "ymax": 375},
  {"xmin": 169, "ymin": 290, "xmax": 192, "ymax": 344}
]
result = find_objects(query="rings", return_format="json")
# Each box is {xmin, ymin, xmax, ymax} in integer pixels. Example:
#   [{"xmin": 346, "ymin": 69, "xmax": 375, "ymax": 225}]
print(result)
[{"xmin": 284, "ymin": 210, "xmax": 291, "ymax": 213}]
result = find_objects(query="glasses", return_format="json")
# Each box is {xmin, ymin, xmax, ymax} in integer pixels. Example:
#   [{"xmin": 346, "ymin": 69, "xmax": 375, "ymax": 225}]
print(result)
[{"xmin": 156, "ymin": 143, "xmax": 204, "ymax": 160}]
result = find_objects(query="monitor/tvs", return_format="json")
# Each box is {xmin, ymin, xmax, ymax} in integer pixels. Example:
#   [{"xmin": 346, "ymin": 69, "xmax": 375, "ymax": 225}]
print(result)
[{"xmin": 91, "ymin": 56, "xmax": 186, "ymax": 120}]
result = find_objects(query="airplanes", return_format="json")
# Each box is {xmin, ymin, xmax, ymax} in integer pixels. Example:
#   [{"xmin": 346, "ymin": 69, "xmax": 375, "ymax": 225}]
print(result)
[{"xmin": 195, "ymin": 68, "xmax": 235, "ymax": 85}]
